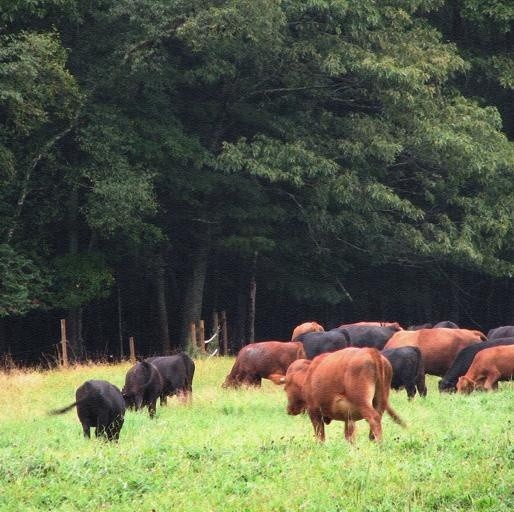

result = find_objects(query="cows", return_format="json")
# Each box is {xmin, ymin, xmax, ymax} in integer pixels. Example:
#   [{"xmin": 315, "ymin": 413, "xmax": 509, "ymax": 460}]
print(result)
[
  {"xmin": 121, "ymin": 348, "xmax": 196, "ymax": 420},
  {"xmin": 222, "ymin": 321, "xmax": 514, "ymax": 397},
  {"xmin": 270, "ymin": 347, "xmax": 409, "ymax": 442},
  {"xmin": 47, "ymin": 377, "xmax": 127, "ymax": 444}
]
